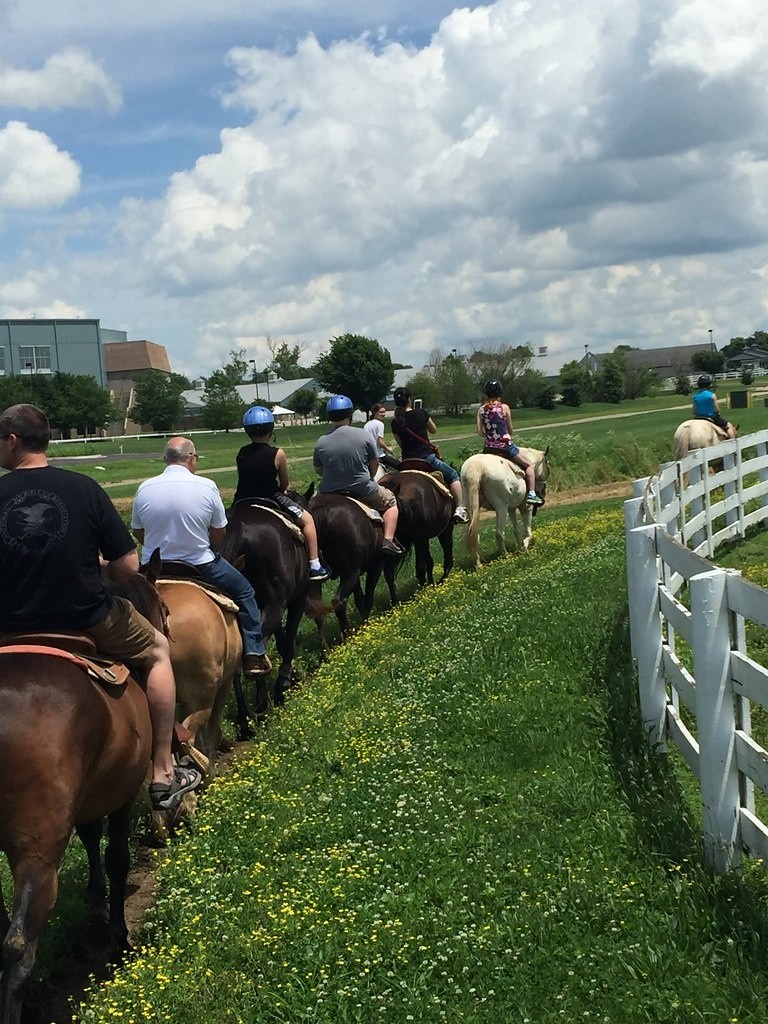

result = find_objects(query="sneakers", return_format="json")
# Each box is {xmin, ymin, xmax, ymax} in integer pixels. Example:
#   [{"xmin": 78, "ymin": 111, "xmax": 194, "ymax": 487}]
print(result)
[{"xmin": 526, "ymin": 493, "xmax": 543, "ymax": 504}]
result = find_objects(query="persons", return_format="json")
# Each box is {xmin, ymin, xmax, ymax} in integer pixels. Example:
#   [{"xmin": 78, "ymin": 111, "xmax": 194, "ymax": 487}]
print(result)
[
  {"xmin": 0, "ymin": 404, "xmax": 201, "ymax": 811},
  {"xmin": 231, "ymin": 406, "xmax": 332, "ymax": 580},
  {"xmin": 313, "ymin": 395, "xmax": 402, "ymax": 553},
  {"xmin": 363, "ymin": 403, "xmax": 401, "ymax": 471},
  {"xmin": 477, "ymin": 379, "xmax": 542, "ymax": 504},
  {"xmin": 391, "ymin": 387, "xmax": 469, "ymax": 524},
  {"xmin": 131, "ymin": 437, "xmax": 273, "ymax": 674},
  {"xmin": 692, "ymin": 375, "xmax": 735, "ymax": 438}
]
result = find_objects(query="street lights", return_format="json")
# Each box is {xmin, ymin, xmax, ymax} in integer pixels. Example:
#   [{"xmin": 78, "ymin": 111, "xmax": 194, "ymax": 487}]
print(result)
[
  {"xmin": 25, "ymin": 363, "xmax": 34, "ymax": 405},
  {"xmin": 585, "ymin": 345, "xmax": 588, "ymax": 361},
  {"xmin": 452, "ymin": 349, "xmax": 457, "ymax": 358},
  {"xmin": 249, "ymin": 360, "xmax": 259, "ymax": 399},
  {"xmin": 708, "ymin": 330, "xmax": 713, "ymax": 352}
]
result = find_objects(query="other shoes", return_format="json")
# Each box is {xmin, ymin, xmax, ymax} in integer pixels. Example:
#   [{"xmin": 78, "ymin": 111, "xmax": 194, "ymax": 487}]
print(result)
[
  {"xmin": 242, "ymin": 654, "xmax": 272, "ymax": 676},
  {"xmin": 453, "ymin": 508, "xmax": 468, "ymax": 522},
  {"xmin": 382, "ymin": 538, "xmax": 403, "ymax": 554},
  {"xmin": 308, "ymin": 566, "xmax": 330, "ymax": 580}
]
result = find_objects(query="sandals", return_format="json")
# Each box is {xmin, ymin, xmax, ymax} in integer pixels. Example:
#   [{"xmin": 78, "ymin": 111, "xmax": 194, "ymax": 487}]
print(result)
[{"xmin": 148, "ymin": 765, "xmax": 202, "ymax": 810}]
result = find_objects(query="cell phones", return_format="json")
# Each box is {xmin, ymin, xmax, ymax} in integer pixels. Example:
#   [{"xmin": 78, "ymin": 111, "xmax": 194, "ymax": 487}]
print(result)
[{"xmin": 413, "ymin": 399, "xmax": 421, "ymax": 410}]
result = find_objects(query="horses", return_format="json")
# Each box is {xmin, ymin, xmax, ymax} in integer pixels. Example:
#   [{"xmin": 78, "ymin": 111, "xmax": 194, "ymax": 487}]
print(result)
[
  {"xmin": 461, "ymin": 445, "xmax": 551, "ymax": 569},
  {"xmin": 210, "ymin": 477, "xmax": 330, "ymax": 727},
  {"xmin": 140, "ymin": 555, "xmax": 252, "ymax": 788},
  {"xmin": 376, "ymin": 463, "xmax": 455, "ymax": 591},
  {"xmin": 305, "ymin": 458, "xmax": 399, "ymax": 660},
  {"xmin": 0, "ymin": 545, "xmax": 174, "ymax": 1024},
  {"xmin": 673, "ymin": 418, "xmax": 741, "ymax": 489}
]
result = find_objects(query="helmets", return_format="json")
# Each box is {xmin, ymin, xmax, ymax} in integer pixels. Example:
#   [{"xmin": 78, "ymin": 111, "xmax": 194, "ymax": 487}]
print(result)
[
  {"xmin": 484, "ymin": 379, "xmax": 504, "ymax": 393},
  {"xmin": 698, "ymin": 375, "xmax": 710, "ymax": 383},
  {"xmin": 242, "ymin": 405, "xmax": 274, "ymax": 434},
  {"xmin": 326, "ymin": 394, "xmax": 354, "ymax": 422}
]
